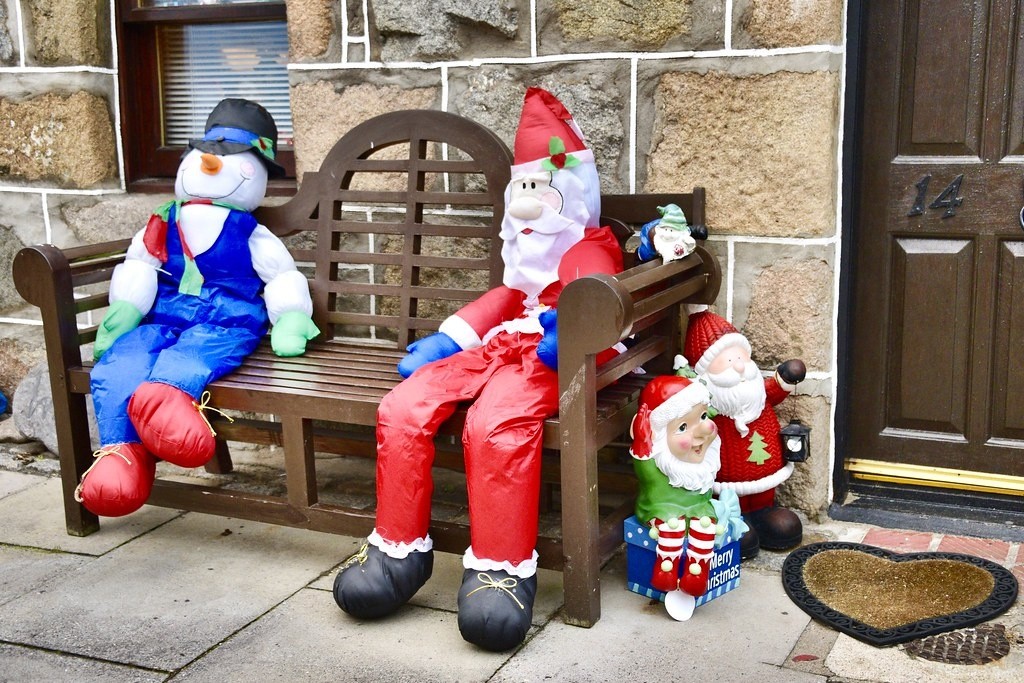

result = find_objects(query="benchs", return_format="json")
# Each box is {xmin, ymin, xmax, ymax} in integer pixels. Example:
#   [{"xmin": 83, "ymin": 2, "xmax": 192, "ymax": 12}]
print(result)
[{"xmin": 11, "ymin": 109, "xmax": 724, "ymax": 629}]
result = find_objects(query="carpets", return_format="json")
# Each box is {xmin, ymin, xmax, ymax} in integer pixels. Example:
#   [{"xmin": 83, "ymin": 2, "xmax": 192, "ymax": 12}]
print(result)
[{"xmin": 782, "ymin": 542, "xmax": 1019, "ymax": 646}]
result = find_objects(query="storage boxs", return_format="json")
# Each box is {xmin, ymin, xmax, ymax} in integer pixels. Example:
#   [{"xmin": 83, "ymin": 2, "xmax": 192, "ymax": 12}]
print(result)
[{"xmin": 624, "ymin": 513, "xmax": 745, "ymax": 610}]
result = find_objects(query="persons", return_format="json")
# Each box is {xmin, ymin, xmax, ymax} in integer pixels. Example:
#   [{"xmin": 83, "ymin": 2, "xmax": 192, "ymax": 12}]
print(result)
[
  {"xmin": 626, "ymin": 203, "xmax": 707, "ymax": 262},
  {"xmin": 630, "ymin": 375, "xmax": 721, "ymax": 620},
  {"xmin": 334, "ymin": 84, "xmax": 624, "ymax": 648},
  {"xmin": 685, "ymin": 311, "xmax": 806, "ymax": 559}
]
